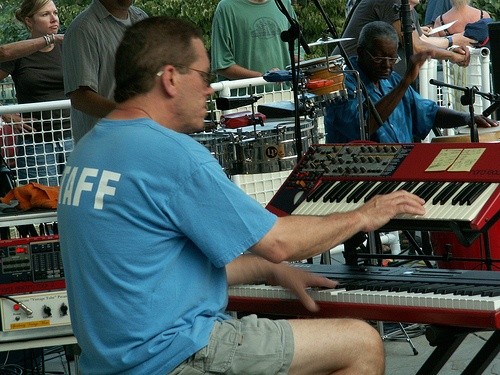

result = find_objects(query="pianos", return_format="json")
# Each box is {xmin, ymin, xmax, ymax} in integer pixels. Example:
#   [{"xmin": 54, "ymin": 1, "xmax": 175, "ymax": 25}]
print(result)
[
  {"xmin": 225, "ymin": 264, "xmax": 500, "ymax": 375},
  {"xmin": 265, "ymin": 143, "xmax": 500, "ymax": 271}
]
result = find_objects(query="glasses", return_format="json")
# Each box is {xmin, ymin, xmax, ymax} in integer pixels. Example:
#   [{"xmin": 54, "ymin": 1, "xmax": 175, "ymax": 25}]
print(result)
[
  {"xmin": 362, "ymin": 50, "xmax": 401, "ymax": 65},
  {"xmin": 157, "ymin": 65, "xmax": 217, "ymax": 84}
]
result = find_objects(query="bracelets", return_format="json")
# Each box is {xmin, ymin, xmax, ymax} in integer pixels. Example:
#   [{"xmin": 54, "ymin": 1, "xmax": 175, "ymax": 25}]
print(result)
[
  {"xmin": 46, "ymin": 34, "xmax": 50, "ymax": 42},
  {"xmin": 44, "ymin": 36, "xmax": 49, "ymax": 45},
  {"xmin": 52, "ymin": 33, "xmax": 55, "ymax": 41}
]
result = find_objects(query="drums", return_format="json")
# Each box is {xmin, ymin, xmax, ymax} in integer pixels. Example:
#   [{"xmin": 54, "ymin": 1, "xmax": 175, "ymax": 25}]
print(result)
[
  {"xmin": 454, "ymin": 121, "xmax": 500, "ymax": 140},
  {"xmin": 431, "ymin": 134, "xmax": 471, "ymax": 144},
  {"xmin": 231, "ymin": 116, "xmax": 318, "ymax": 175},
  {"xmin": 188, "ymin": 131, "xmax": 232, "ymax": 180},
  {"xmin": 285, "ymin": 55, "xmax": 348, "ymax": 110}
]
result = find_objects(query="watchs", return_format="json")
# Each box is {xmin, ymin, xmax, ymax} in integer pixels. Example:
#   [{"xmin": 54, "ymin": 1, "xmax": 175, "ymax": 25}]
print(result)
[{"xmin": 445, "ymin": 36, "xmax": 453, "ymax": 47}]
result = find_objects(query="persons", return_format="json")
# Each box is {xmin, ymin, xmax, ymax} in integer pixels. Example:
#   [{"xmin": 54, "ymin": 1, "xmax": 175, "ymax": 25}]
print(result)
[
  {"xmin": 62, "ymin": 0, "xmax": 149, "ymax": 145},
  {"xmin": 0, "ymin": 113, "xmax": 39, "ymax": 257},
  {"xmin": 211, "ymin": 0, "xmax": 305, "ymax": 97},
  {"xmin": 324, "ymin": 21, "xmax": 500, "ymax": 144},
  {"xmin": 0, "ymin": 0, "xmax": 78, "ymax": 186},
  {"xmin": 329, "ymin": 0, "xmax": 496, "ymax": 106},
  {"xmin": 57, "ymin": 15, "xmax": 426, "ymax": 375}
]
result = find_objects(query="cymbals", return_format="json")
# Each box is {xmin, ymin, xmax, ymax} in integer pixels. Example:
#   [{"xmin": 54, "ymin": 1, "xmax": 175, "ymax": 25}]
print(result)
[{"xmin": 299, "ymin": 37, "xmax": 355, "ymax": 47}]
